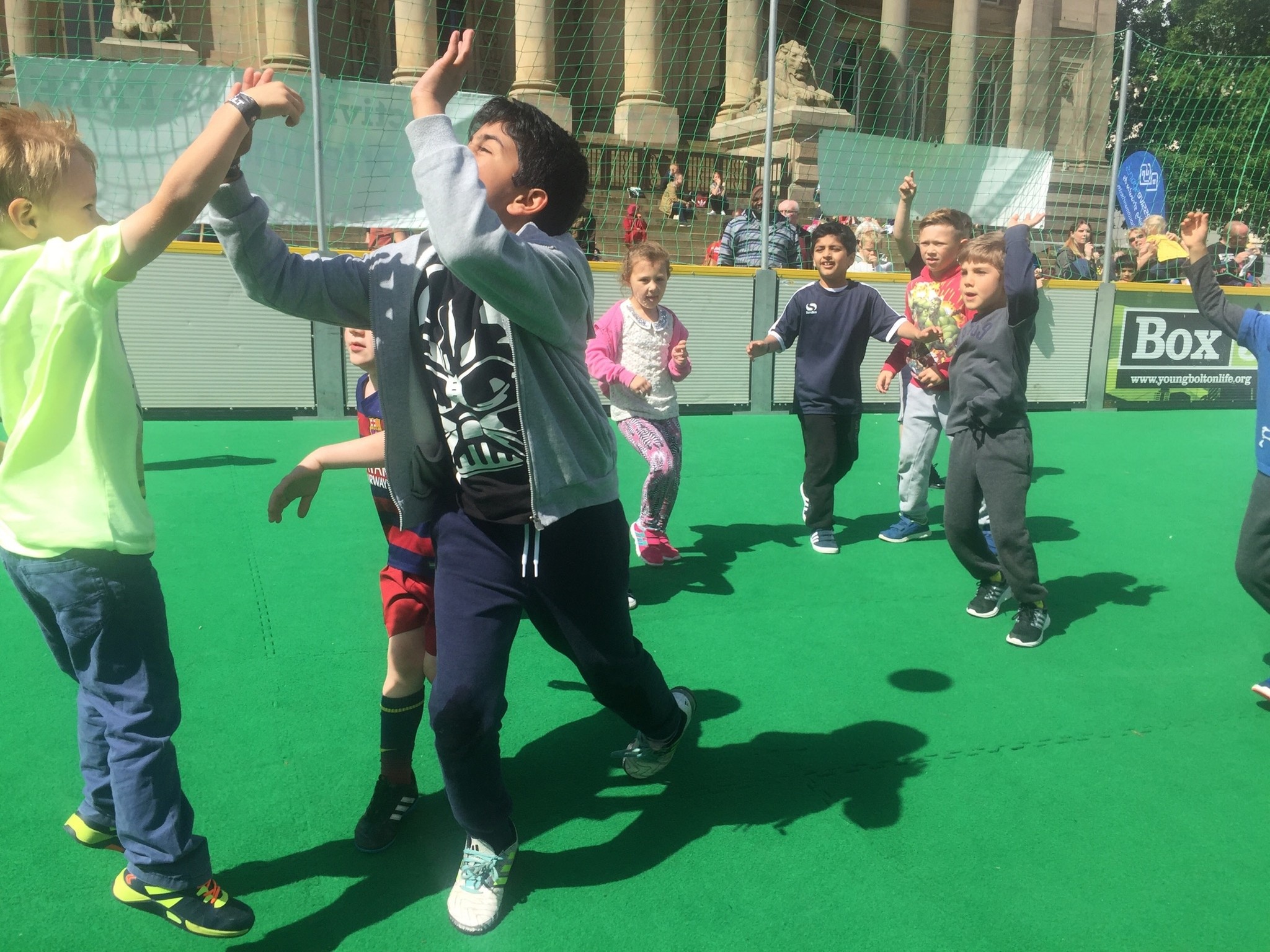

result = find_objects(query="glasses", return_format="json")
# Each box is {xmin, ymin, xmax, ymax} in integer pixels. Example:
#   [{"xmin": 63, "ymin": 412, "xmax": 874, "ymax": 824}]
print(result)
[
  {"xmin": 789, "ymin": 211, "xmax": 799, "ymax": 213},
  {"xmin": 1129, "ymin": 234, "xmax": 1144, "ymax": 243},
  {"xmin": 1122, "ymin": 270, "xmax": 1133, "ymax": 273},
  {"xmin": 1238, "ymin": 233, "xmax": 1249, "ymax": 240}
]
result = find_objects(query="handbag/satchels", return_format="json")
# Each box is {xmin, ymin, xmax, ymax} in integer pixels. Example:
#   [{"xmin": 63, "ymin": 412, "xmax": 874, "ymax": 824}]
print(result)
[
  {"xmin": 629, "ymin": 189, "xmax": 646, "ymax": 199},
  {"xmin": 695, "ymin": 192, "xmax": 708, "ymax": 208}
]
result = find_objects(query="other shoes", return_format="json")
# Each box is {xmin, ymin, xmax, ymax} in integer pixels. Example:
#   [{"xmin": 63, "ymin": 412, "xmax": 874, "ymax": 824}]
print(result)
[
  {"xmin": 929, "ymin": 463, "xmax": 946, "ymax": 489},
  {"xmin": 707, "ymin": 209, "xmax": 716, "ymax": 215},
  {"xmin": 720, "ymin": 211, "xmax": 726, "ymax": 215},
  {"xmin": 679, "ymin": 223, "xmax": 692, "ymax": 227},
  {"xmin": 1251, "ymin": 678, "xmax": 1270, "ymax": 699}
]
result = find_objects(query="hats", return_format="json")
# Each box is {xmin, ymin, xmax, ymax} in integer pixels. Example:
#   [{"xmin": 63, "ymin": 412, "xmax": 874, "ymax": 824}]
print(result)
[{"xmin": 854, "ymin": 221, "xmax": 889, "ymax": 239}]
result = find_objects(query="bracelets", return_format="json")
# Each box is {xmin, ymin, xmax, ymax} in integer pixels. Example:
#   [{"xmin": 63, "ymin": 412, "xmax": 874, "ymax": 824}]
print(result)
[
  {"xmin": 226, "ymin": 93, "xmax": 261, "ymax": 132},
  {"xmin": 223, "ymin": 157, "xmax": 242, "ymax": 178}
]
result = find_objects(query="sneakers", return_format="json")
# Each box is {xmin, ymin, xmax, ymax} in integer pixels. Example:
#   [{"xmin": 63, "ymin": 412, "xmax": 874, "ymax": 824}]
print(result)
[
  {"xmin": 63, "ymin": 812, "xmax": 128, "ymax": 855},
  {"xmin": 1005, "ymin": 602, "xmax": 1052, "ymax": 648},
  {"xmin": 660, "ymin": 533, "xmax": 681, "ymax": 561},
  {"xmin": 630, "ymin": 521, "xmax": 665, "ymax": 565},
  {"xmin": 966, "ymin": 570, "xmax": 1013, "ymax": 618},
  {"xmin": 622, "ymin": 686, "xmax": 696, "ymax": 781},
  {"xmin": 799, "ymin": 482, "xmax": 809, "ymax": 522},
  {"xmin": 445, "ymin": 818, "xmax": 520, "ymax": 934},
  {"xmin": 810, "ymin": 528, "xmax": 839, "ymax": 554},
  {"xmin": 355, "ymin": 767, "xmax": 419, "ymax": 853},
  {"xmin": 982, "ymin": 524, "xmax": 997, "ymax": 557},
  {"xmin": 114, "ymin": 866, "xmax": 255, "ymax": 937},
  {"xmin": 628, "ymin": 592, "xmax": 636, "ymax": 610},
  {"xmin": 877, "ymin": 511, "xmax": 932, "ymax": 543}
]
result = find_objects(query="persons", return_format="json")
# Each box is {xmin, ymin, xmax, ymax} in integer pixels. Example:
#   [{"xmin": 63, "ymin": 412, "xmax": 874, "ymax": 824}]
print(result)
[
  {"xmin": 266, "ymin": 232, "xmax": 442, "ymax": 851},
  {"xmin": 202, "ymin": 29, "xmax": 695, "ymax": 937},
  {"xmin": 893, "ymin": 171, "xmax": 946, "ymax": 489},
  {"xmin": 744, "ymin": 222, "xmax": 942, "ymax": 555},
  {"xmin": 877, "ymin": 209, "xmax": 1000, "ymax": 556},
  {"xmin": 584, "ymin": 242, "xmax": 691, "ymax": 568},
  {"xmin": 0, "ymin": 69, "xmax": 307, "ymax": 942},
  {"xmin": 942, "ymin": 211, "xmax": 1052, "ymax": 649},
  {"xmin": 1181, "ymin": 211, "xmax": 1270, "ymax": 705},
  {"xmin": 568, "ymin": 160, "xmax": 1270, "ymax": 290},
  {"xmin": 363, "ymin": 227, "xmax": 409, "ymax": 252}
]
